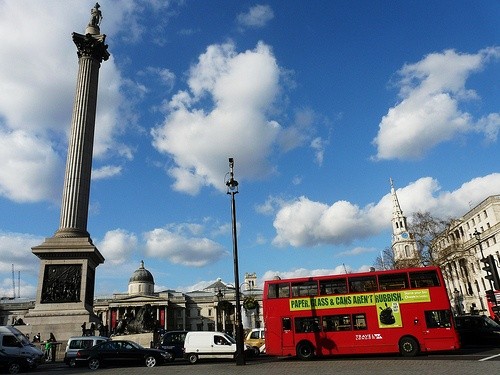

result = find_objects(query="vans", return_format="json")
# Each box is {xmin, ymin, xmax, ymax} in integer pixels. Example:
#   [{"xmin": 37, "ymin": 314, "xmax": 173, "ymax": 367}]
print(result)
[
  {"xmin": 154, "ymin": 330, "xmax": 189, "ymax": 362},
  {"xmin": 0, "ymin": 326, "xmax": 46, "ymax": 366},
  {"xmin": 245, "ymin": 327, "xmax": 265, "ymax": 358},
  {"xmin": 63, "ymin": 336, "xmax": 111, "ymax": 366},
  {"xmin": 184, "ymin": 331, "xmax": 260, "ymax": 365}
]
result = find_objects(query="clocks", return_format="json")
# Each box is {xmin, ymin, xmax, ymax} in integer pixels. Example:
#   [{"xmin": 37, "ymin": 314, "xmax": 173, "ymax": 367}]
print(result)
[{"xmin": 402, "ymin": 232, "xmax": 409, "ymax": 239}]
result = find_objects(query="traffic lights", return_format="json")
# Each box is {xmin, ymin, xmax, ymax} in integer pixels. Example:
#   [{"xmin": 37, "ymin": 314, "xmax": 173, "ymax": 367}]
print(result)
[
  {"xmin": 484, "ymin": 289, "xmax": 497, "ymax": 303},
  {"xmin": 479, "ymin": 254, "xmax": 500, "ymax": 291}
]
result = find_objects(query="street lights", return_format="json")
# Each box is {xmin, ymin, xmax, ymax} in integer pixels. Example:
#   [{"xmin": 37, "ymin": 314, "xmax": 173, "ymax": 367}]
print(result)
[
  {"xmin": 225, "ymin": 157, "xmax": 246, "ymax": 366},
  {"xmin": 473, "ymin": 228, "xmax": 500, "ymax": 316}
]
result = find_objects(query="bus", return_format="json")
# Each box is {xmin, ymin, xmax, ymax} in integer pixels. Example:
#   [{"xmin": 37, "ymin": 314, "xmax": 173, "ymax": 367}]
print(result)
[{"xmin": 262, "ymin": 265, "xmax": 462, "ymax": 361}]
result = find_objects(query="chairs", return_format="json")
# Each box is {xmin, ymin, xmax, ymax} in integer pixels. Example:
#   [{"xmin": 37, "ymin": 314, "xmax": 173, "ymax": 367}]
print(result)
[
  {"xmin": 350, "ymin": 286, "xmax": 359, "ymax": 292},
  {"xmin": 321, "ymin": 288, "xmax": 327, "ymax": 294},
  {"xmin": 334, "ymin": 289, "xmax": 340, "ymax": 293}
]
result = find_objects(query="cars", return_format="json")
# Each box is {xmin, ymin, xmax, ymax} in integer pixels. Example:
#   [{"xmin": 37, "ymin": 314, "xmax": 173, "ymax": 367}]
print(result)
[
  {"xmin": 0, "ymin": 349, "xmax": 37, "ymax": 375},
  {"xmin": 441, "ymin": 315, "xmax": 500, "ymax": 355},
  {"xmin": 71, "ymin": 339, "xmax": 174, "ymax": 370}
]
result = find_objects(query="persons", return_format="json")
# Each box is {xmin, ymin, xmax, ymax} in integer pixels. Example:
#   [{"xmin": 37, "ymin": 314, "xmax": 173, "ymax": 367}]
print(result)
[
  {"xmin": 216, "ymin": 336, "xmax": 226, "ymax": 345},
  {"xmin": 44, "ymin": 340, "xmax": 51, "ymax": 359},
  {"xmin": 50, "ymin": 333, "xmax": 57, "ymax": 361}
]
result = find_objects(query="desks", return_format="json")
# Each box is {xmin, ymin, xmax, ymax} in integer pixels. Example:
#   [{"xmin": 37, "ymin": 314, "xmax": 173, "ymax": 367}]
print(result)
[{"xmin": 40, "ymin": 342, "xmax": 63, "ymax": 361}]
